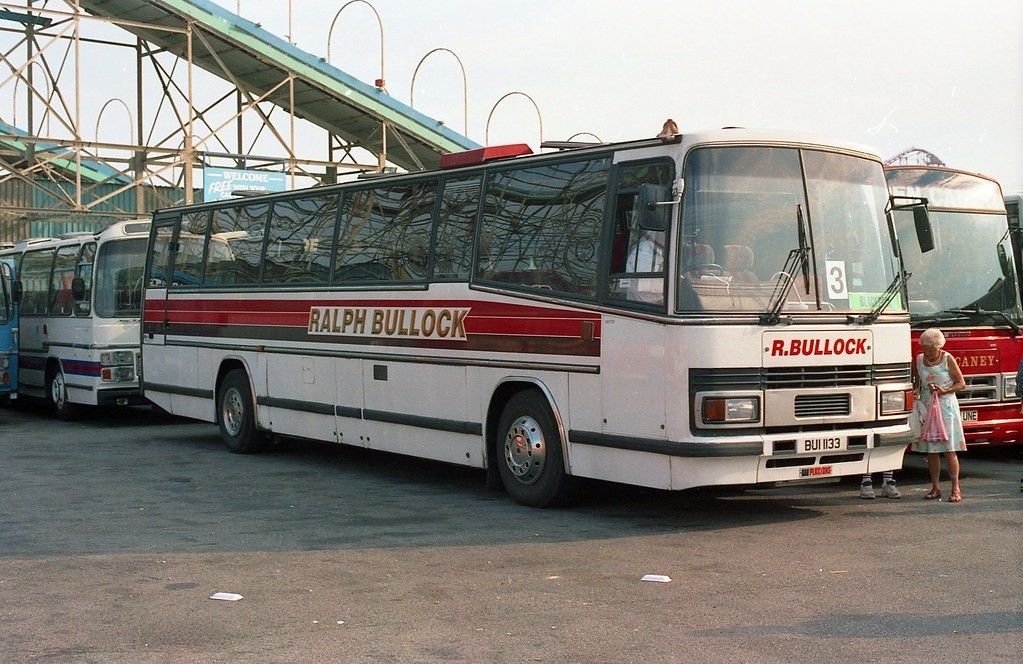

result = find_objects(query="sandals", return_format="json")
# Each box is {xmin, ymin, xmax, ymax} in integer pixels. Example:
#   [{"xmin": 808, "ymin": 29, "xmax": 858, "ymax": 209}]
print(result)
[
  {"xmin": 949, "ymin": 492, "xmax": 961, "ymax": 502},
  {"xmin": 926, "ymin": 489, "xmax": 940, "ymax": 499}
]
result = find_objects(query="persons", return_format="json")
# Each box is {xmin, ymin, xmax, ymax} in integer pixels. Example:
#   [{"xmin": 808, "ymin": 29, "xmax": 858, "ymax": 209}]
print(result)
[
  {"xmin": 860, "ymin": 471, "xmax": 901, "ymax": 499},
  {"xmin": 1015, "ymin": 358, "xmax": 1023, "ymax": 492},
  {"xmin": 626, "ymin": 229, "xmax": 683, "ymax": 307},
  {"xmin": 908, "ymin": 327, "xmax": 966, "ymax": 502}
]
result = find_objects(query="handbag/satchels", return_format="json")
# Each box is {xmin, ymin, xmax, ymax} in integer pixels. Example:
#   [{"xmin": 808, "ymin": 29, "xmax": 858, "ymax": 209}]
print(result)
[{"xmin": 921, "ymin": 390, "xmax": 948, "ymax": 442}]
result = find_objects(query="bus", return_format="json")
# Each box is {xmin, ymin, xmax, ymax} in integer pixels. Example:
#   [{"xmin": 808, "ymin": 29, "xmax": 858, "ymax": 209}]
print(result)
[
  {"xmin": 139, "ymin": 118, "xmax": 936, "ymax": 511},
  {"xmin": 621, "ymin": 150, "xmax": 1023, "ymax": 463},
  {"xmin": 0, "ymin": 214, "xmax": 317, "ymax": 424}
]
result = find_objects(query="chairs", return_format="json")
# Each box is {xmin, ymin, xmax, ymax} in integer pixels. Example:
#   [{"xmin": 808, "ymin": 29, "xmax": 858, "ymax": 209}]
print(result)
[{"xmin": 682, "ymin": 245, "xmax": 760, "ymax": 285}]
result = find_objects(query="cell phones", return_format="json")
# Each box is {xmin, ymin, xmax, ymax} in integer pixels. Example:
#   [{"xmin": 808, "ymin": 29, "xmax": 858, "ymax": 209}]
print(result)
[{"xmin": 929, "ymin": 383, "xmax": 937, "ymax": 390}]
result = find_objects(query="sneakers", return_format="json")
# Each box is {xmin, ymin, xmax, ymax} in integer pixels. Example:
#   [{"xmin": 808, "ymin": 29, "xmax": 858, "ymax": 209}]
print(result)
[
  {"xmin": 881, "ymin": 479, "xmax": 901, "ymax": 498},
  {"xmin": 860, "ymin": 481, "xmax": 875, "ymax": 499}
]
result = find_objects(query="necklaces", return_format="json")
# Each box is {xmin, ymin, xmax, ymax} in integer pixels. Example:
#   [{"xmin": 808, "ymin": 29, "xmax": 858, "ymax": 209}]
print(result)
[{"xmin": 927, "ymin": 349, "xmax": 942, "ymax": 367}]
